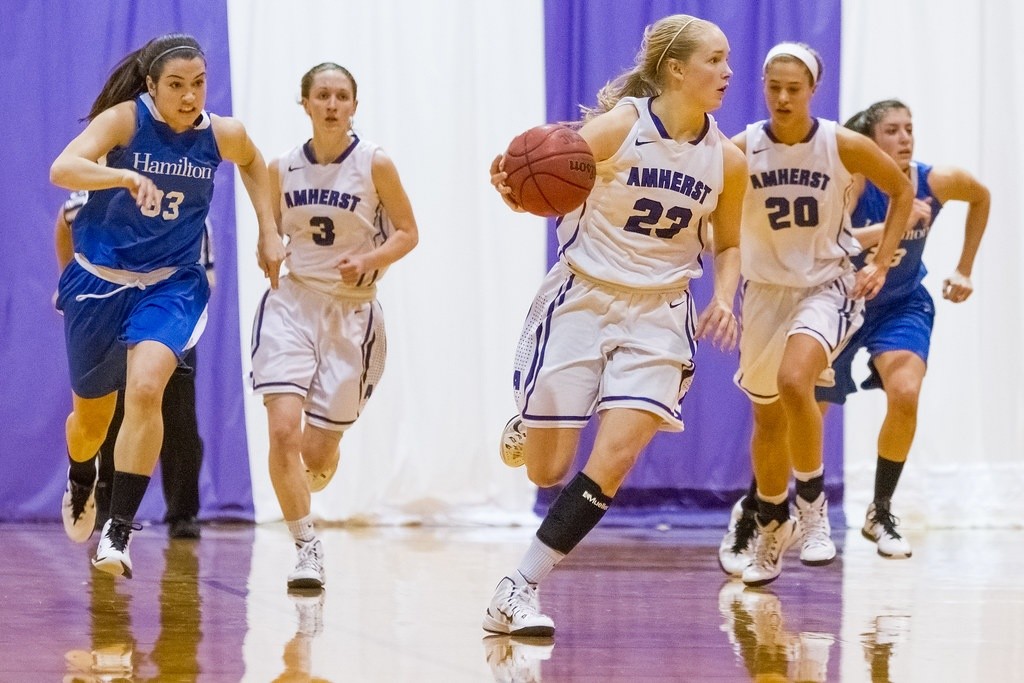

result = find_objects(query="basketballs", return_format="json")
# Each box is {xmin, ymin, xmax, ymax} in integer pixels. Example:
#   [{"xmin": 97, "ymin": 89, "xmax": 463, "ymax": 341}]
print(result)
[{"xmin": 502, "ymin": 124, "xmax": 597, "ymax": 218}]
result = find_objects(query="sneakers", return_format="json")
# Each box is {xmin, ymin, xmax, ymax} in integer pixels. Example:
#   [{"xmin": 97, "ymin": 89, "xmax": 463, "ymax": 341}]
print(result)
[
  {"xmin": 500, "ymin": 414, "xmax": 529, "ymax": 468},
  {"xmin": 484, "ymin": 637, "xmax": 556, "ymax": 683},
  {"xmin": 718, "ymin": 586, "xmax": 800, "ymax": 676},
  {"xmin": 861, "ymin": 496, "xmax": 912, "ymax": 560},
  {"xmin": 62, "ymin": 450, "xmax": 103, "ymax": 543},
  {"xmin": 91, "ymin": 515, "xmax": 144, "ymax": 580},
  {"xmin": 742, "ymin": 513, "xmax": 801, "ymax": 586},
  {"xmin": 718, "ymin": 495, "xmax": 759, "ymax": 577},
  {"xmin": 482, "ymin": 569, "xmax": 555, "ymax": 636},
  {"xmin": 290, "ymin": 594, "xmax": 326, "ymax": 637},
  {"xmin": 795, "ymin": 490, "xmax": 837, "ymax": 565},
  {"xmin": 861, "ymin": 607, "xmax": 909, "ymax": 664},
  {"xmin": 303, "ymin": 463, "xmax": 336, "ymax": 493},
  {"xmin": 288, "ymin": 537, "xmax": 326, "ymax": 588}
]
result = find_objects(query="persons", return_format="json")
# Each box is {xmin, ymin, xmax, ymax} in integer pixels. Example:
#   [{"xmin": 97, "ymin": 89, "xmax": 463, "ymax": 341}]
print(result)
[
  {"xmin": 482, "ymin": 15, "xmax": 748, "ymax": 638},
  {"xmin": 705, "ymin": 42, "xmax": 912, "ymax": 588},
  {"xmin": 249, "ymin": 62, "xmax": 418, "ymax": 590},
  {"xmin": 718, "ymin": 98, "xmax": 989, "ymax": 575},
  {"xmin": 53, "ymin": 33, "xmax": 288, "ymax": 581}
]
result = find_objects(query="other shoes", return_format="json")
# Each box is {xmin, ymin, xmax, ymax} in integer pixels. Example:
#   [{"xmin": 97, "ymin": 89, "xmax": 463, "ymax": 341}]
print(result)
[
  {"xmin": 96, "ymin": 487, "xmax": 111, "ymax": 532},
  {"xmin": 168, "ymin": 514, "xmax": 200, "ymax": 537}
]
